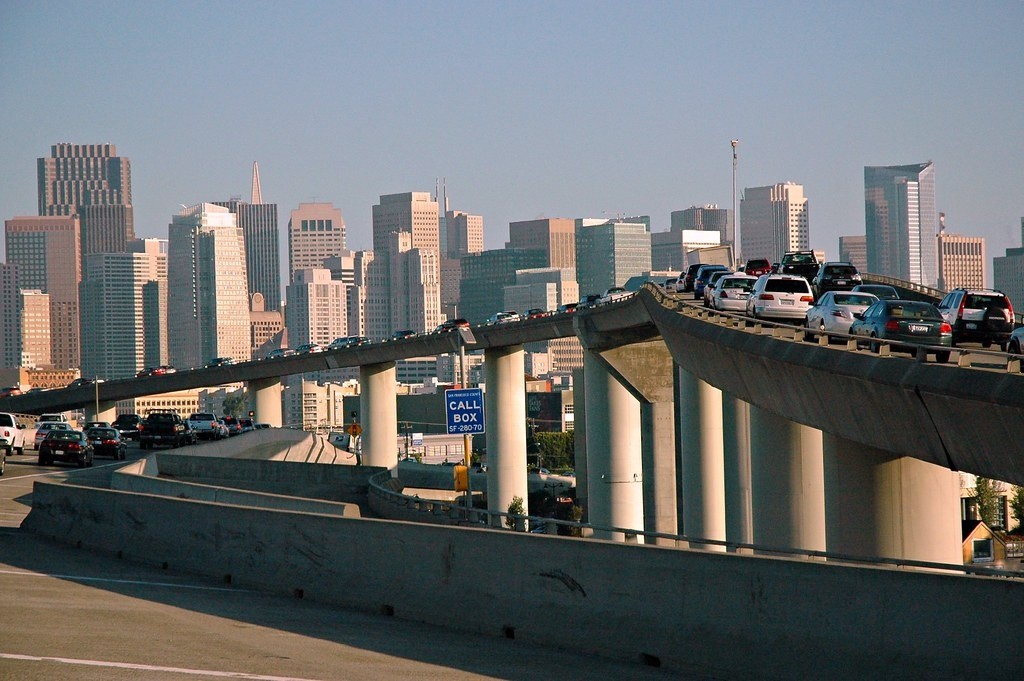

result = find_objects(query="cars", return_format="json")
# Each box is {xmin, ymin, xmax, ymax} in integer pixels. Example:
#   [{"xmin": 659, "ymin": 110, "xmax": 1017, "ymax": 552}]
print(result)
[
  {"xmin": 0, "ymin": 387, "xmax": 24, "ymax": 399},
  {"xmin": 37, "ymin": 430, "xmax": 95, "ymax": 468},
  {"xmin": 159, "ymin": 364, "xmax": 177, "ymax": 374},
  {"xmin": 852, "ymin": 284, "xmax": 900, "ymax": 310},
  {"xmin": 204, "ymin": 356, "xmax": 237, "ymax": 369},
  {"xmin": 530, "ymin": 467, "xmax": 551, "ymax": 474},
  {"xmin": 744, "ymin": 274, "xmax": 815, "ymax": 326},
  {"xmin": 134, "ymin": 366, "xmax": 165, "ymax": 378},
  {"xmin": 85, "ymin": 426, "xmax": 127, "ymax": 461},
  {"xmin": 265, "ymin": 286, "xmax": 633, "ymax": 359},
  {"xmin": 848, "ymin": 299, "xmax": 953, "ymax": 363},
  {"xmin": 657, "ymin": 257, "xmax": 780, "ymax": 307},
  {"xmin": 67, "ymin": 377, "xmax": 92, "ymax": 388},
  {"xmin": 26, "ymin": 386, "xmax": 49, "ymax": 394},
  {"xmin": 561, "ymin": 471, "xmax": 576, "ymax": 477},
  {"xmin": 82, "ymin": 421, "xmax": 112, "ymax": 433},
  {"xmin": 110, "ymin": 408, "xmax": 297, "ymax": 450},
  {"xmin": 34, "ymin": 420, "xmax": 74, "ymax": 452},
  {"xmin": 1004, "ymin": 316, "xmax": 1024, "ymax": 371},
  {"xmin": 803, "ymin": 291, "xmax": 883, "ymax": 342},
  {"xmin": 708, "ymin": 272, "xmax": 758, "ymax": 315},
  {"xmin": 0, "ymin": 435, "xmax": 10, "ymax": 475}
]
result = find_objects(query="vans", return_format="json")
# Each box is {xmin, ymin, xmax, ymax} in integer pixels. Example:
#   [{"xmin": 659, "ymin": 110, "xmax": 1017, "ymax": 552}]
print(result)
[{"xmin": 0, "ymin": 412, "xmax": 27, "ymax": 456}]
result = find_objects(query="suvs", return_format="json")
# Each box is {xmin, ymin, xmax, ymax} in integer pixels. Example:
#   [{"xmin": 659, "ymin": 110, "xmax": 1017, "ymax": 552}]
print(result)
[
  {"xmin": 812, "ymin": 260, "xmax": 864, "ymax": 303},
  {"xmin": 33, "ymin": 412, "xmax": 69, "ymax": 428},
  {"xmin": 933, "ymin": 287, "xmax": 1016, "ymax": 352}
]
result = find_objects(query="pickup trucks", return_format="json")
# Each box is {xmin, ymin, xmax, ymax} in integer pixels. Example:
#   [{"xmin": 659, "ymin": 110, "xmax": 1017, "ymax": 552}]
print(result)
[{"xmin": 772, "ymin": 248, "xmax": 822, "ymax": 284}]
